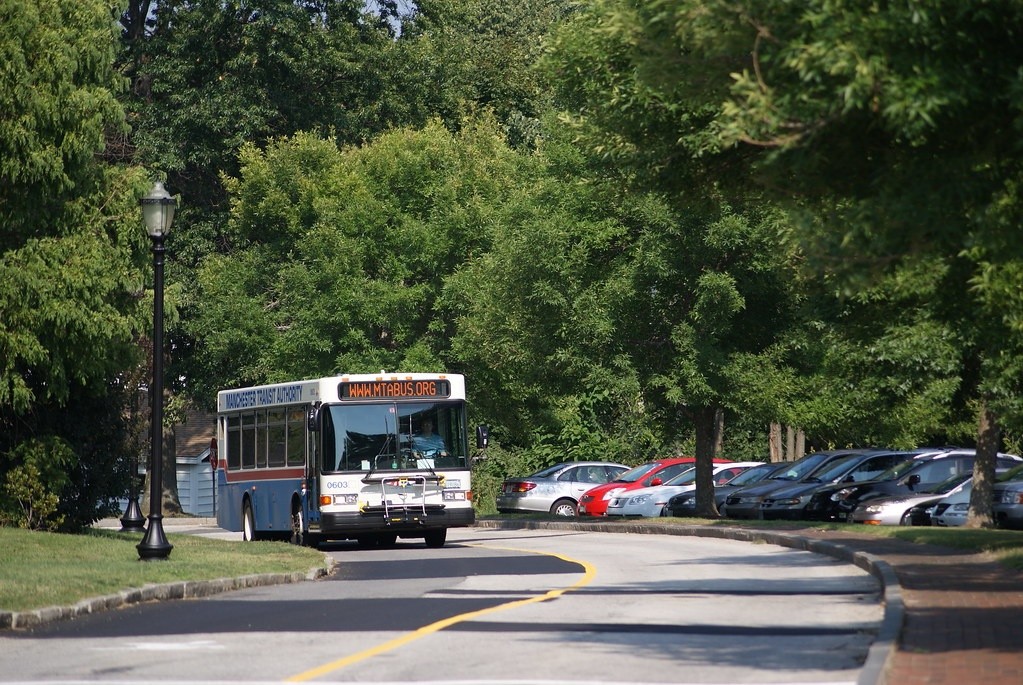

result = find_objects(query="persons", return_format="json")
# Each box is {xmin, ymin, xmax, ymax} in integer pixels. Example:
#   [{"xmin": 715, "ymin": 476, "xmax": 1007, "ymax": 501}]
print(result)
[{"xmin": 412, "ymin": 418, "xmax": 447, "ymax": 458}]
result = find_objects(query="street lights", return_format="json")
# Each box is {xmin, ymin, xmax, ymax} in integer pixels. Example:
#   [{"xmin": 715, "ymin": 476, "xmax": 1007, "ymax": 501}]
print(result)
[{"xmin": 136, "ymin": 181, "xmax": 177, "ymax": 560}]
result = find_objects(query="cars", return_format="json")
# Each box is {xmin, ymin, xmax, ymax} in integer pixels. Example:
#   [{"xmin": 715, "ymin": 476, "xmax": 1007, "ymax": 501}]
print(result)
[
  {"xmin": 930, "ymin": 456, "xmax": 1023, "ymax": 530},
  {"xmin": 662, "ymin": 461, "xmax": 794, "ymax": 519},
  {"xmin": 855, "ymin": 469, "xmax": 1011, "ymax": 526},
  {"xmin": 577, "ymin": 457, "xmax": 744, "ymax": 518},
  {"xmin": 725, "ymin": 449, "xmax": 876, "ymax": 519},
  {"xmin": 607, "ymin": 460, "xmax": 765, "ymax": 519},
  {"xmin": 762, "ymin": 449, "xmax": 927, "ymax": 517},
  {"xmin": 496, "ymin": 461, "xmax": 631, "ymax": 516}
]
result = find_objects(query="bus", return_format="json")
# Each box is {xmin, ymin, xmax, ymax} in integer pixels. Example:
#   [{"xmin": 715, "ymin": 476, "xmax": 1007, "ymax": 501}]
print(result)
[{"xmin": 214, "ymin": 372, "xmax": 488, "ymax": 549}]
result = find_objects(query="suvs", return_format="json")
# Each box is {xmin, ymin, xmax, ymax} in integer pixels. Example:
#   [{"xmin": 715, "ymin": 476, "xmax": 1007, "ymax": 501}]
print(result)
[{"xmin": 806, "ymin": 449, "xmax": 1023, "ymax": 521}]
